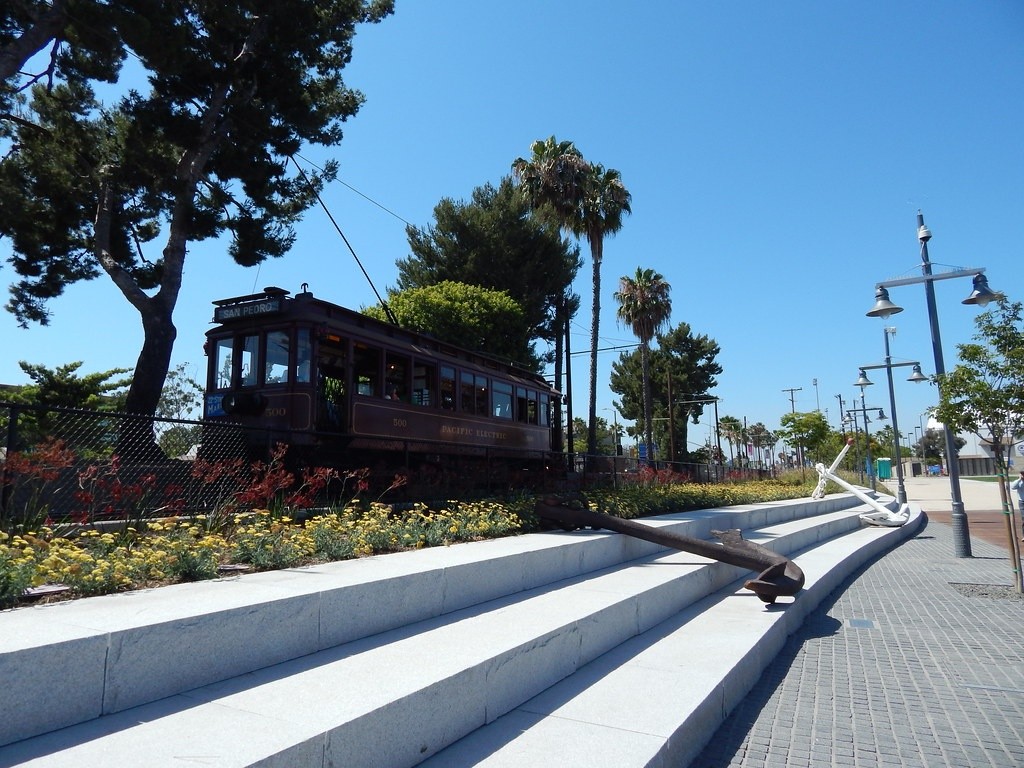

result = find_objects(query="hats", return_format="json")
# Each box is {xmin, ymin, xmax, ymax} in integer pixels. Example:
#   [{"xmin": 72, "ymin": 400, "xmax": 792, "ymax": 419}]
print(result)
[{"xmin": 1019, "ymin": 467, "xmax": 1024, "ymax": 472}]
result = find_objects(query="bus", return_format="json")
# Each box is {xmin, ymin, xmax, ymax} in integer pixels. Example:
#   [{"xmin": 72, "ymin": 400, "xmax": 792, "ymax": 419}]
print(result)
[{"xmin": 198, "ymin": 154, "xmax": 562, "ymax": 490}]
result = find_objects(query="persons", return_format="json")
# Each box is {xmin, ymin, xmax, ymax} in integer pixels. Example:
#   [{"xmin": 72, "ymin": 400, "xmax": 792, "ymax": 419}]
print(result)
[{"xmin": 1011, "ymin": 470, "xmax": 1024, "ymax": 541}]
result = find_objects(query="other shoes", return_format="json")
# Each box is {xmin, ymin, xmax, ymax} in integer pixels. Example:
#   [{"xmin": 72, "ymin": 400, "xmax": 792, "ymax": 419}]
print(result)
[{"xmin": 1021, "ymin": 538, "xmax": 1024, "ymax": 542}]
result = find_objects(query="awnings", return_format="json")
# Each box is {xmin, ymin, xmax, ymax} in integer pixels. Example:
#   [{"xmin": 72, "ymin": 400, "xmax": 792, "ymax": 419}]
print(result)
[{"xmin": 978, "ymin": 437, "xmax": 1015, "ymax": 446}]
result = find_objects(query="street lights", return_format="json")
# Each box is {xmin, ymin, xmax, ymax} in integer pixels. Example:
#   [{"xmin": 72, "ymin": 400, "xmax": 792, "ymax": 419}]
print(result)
[
  {"xmin": 914, "ymin": 426, "xmax": 920, "ymax": 461},
  {"xmin": 866, "ymin": 208, "xmax": 1000, "ymax": 558},
  {"xmin": 853, "ymin": 326, "xmax": 929, "ymax": 510},
  {"xmin": 903, "ymin": 433, "xmax": 913, "ymax": 462},
  {"xmin": 835, "ymin": 388, "xmax": 889, "ymax": 493}
]
